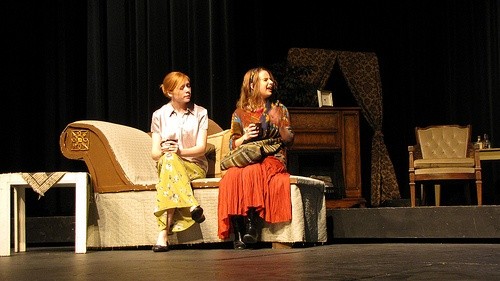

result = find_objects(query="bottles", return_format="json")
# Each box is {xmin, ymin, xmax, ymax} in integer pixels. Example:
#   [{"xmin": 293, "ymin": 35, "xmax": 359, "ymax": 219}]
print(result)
[{"xmin": 475, "ymin": 134, "xmax": 491, "ymax": 149}]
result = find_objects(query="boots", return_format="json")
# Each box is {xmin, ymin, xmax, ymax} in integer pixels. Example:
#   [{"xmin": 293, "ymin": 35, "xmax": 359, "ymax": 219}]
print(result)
[
  {"xmin": 242, "ymin": 208, "xmax": 258, "ymax": 243},
  {"xmin": 231, "ymin": 214, "xmax": 247, "ymax": 249}
]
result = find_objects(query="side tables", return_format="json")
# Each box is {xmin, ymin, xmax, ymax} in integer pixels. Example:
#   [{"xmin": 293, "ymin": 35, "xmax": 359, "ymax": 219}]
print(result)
[{"xmin": 0, "ymin": 172, "xmax": 94, "ymax": 256}]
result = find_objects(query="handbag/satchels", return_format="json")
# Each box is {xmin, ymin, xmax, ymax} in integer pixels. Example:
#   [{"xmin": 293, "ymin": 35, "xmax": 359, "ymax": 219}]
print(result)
[{"xmin": 220, "ymin": 137, "xmax": 283, "ymax": 171}]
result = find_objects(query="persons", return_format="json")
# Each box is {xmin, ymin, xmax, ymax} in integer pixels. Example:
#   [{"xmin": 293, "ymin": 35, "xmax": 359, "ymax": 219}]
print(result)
[
  {"xmin": 151, "ymin": 72, "xmax": 208, "ymax": 252},
  {"xmin": 217, "ymin": 67, "xmax": 294, "ymax": 250}
]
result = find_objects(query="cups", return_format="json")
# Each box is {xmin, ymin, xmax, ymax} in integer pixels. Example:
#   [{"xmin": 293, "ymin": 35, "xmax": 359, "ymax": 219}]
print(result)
[{"xmin": 255, "ymin": 122, "xmax": 262, "ymax": 137}]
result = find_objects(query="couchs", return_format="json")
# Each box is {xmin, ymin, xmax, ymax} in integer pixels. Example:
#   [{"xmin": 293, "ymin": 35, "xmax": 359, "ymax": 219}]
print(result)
[{"xmin": 59, "ymin": 118, "xmax": 329, "ymax": 249}]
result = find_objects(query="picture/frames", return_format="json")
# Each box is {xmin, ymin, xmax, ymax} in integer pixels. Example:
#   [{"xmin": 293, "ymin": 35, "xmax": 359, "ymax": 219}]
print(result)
[{"xmin": 317, "ymin": 89, "xmax": 334, "ymax": 108}]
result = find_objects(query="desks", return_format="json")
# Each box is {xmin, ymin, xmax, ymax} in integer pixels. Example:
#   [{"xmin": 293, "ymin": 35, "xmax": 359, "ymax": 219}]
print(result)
[
  {"xmin": 478, "ymin": 148, "xmax": 500, "ymax": 160},
  {"xmin": 286, "ymin": 107, "xmax": 365, "ymax": 208}
]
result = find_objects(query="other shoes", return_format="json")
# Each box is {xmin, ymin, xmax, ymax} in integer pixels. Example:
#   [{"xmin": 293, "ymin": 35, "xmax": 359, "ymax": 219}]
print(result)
[
  {"xmin": 152, "ymin": 241, "xmax": 168, "ymax": 252},
  {"xmin": 192, "ymin": 206, "xmax": 206, "ymax": 224}
]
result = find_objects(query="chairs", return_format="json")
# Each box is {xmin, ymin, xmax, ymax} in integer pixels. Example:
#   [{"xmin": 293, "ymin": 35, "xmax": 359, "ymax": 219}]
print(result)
[{"xmin": 407, "ymin": 124, "xmax": 482, "ymax": 207}]
